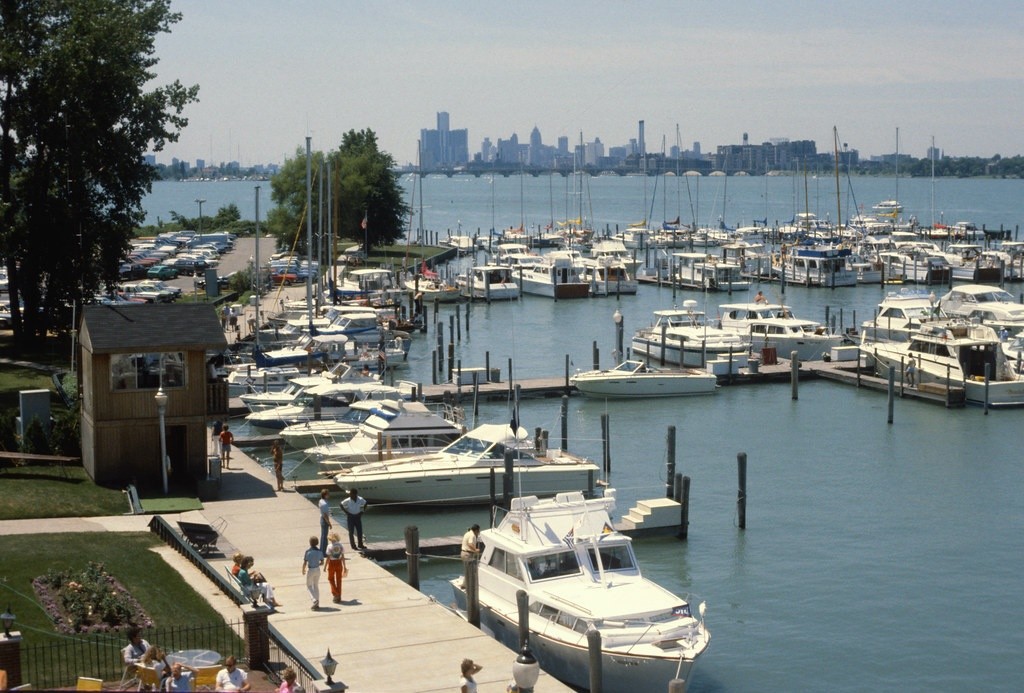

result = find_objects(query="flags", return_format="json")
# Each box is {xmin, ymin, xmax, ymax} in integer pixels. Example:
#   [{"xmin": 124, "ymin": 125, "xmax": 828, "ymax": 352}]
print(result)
[
  {"xmin": 361, "ymin": 218, "xmax": 366, "ymax": 229},
  {"xmin": 672, "ymin": 604, "xmax": 692, "ymax": 618}
]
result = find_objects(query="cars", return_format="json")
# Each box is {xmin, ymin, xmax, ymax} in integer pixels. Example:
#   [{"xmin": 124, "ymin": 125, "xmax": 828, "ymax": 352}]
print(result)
[
  {"xmin": 118, "ymin": 229, "xmax": 237, "ymax": 282},
  {"xmin": 83, "ymin": 280, "xmax": 181, "ymax": 306},
  {"xmin": 0, "ymin": 265, "xmax": 58, "ymax": 330}
]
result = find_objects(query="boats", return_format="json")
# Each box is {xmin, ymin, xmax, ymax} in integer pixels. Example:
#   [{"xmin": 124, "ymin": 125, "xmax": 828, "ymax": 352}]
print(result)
[
  {"xmin": 238, "ymin": 362, "xmax": 470, "ymax": 472},
  {"xmin": 858, "ymin": 284, "xmax": 1024, "ymax": 403},
  {"xmin": 631, "ymin": 300, "xmax": 845, "ymax": 366},
  {"xmin": 448, "ymin": 488, "xmax": 712, "ymax": 693},
  {"xmin": 333, "ymin": 423, "xmax": 602, "ymax": 507},
  {"xmin": 569, "ymin": 360, "xmax": 718, "ymax": 399}
]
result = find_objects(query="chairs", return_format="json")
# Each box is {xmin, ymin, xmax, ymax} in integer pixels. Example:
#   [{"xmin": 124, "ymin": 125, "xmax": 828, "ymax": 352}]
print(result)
[
  {"xmin": 119, "ymin": 647, "xmax": 223, "ymax": 692},
  {"xmin": 77, "ymin": 676, "xmax": 103, "ymax": 691}
]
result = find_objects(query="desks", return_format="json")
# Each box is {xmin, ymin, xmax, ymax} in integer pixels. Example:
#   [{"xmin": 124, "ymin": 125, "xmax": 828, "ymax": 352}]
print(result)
[{"xmin": 166, "ymin": 650, "xmax": 221, "ymax": 668}]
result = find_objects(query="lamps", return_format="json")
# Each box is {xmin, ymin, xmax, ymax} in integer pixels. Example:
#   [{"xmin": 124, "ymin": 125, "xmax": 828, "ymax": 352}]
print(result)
[
  {"xmin": 0, "ymin": 604, "xmax": 16, "ymax": 639},
  {"xmin": 247, "ymin": 579, "xmax": 261, "ymax": 607},
  {"xmin": 319, "ymin": 648, "xmax": 339, "ymax": 685}
]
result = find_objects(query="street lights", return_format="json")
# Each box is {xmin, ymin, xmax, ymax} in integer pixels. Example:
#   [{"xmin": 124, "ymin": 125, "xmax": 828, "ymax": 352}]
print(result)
[
  {"xmin": 194, "ymin": 198, "xmax": 206, "ymax": 245},
  {"xmin": 155, "ymin": 386, "xmax": 168, "ymax": 494}
]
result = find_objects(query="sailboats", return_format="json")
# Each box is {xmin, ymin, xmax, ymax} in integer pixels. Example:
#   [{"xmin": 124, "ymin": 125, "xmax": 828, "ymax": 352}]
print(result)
[
  {"xmin": 439, "ymin": 123, "xmax": 1024, "ymax": 301},
  {"xmin": 224, "ymin": 136, "xmax": 462, "ymax": 386}
]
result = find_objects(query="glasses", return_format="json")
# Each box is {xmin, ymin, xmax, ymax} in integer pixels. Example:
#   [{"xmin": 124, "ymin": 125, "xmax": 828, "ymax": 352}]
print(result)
[{"xmin": 225, "ymin": 664, "xmax": 234, "ymax": 667}]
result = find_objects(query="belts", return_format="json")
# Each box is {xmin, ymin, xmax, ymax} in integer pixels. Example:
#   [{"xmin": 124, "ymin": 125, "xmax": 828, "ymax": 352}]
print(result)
[{"xmin": 463, "ymin": 550, "xmax": 473, "ymax": 553}]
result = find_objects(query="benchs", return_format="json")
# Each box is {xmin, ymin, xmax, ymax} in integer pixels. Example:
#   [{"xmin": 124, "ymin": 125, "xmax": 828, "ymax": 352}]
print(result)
[{"xmin": 224, "ymin": 565, "xmax": 264, "ymax": 604}]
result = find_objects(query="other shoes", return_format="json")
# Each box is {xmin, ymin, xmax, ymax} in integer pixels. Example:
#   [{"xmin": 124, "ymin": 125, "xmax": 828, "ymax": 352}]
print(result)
[
  {"xmin": 270, "ymin": 601, "xmax": 279, "ymax": 607},
  {"xmin": 311, "ymin": 602, "xmax": 318, "ymax": 611},
  {"xmin": 333, "ymin": 596, "xmax": 341, "ymax": 603},
  {"xmin": 352, "ymin": 546, "xmax": 359, "ymax": 550},
  {"xmin": 358, "ymin": 544, "xmax": 367, "ymax": 549}
]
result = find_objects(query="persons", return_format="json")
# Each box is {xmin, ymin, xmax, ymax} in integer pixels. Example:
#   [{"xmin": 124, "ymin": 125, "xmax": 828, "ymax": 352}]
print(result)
[
  {"xmin": 905, "ymin": 353, "xmax": 916, "ymax": 388},
  {"xmin": 998, "ymin": 326, "xmax": 1008, "ymax": 342},
  {"xmin": 278, "ymin": 667, "xmax": 305, "ymax": 693},
  {"xmin": 141, "ymin": 646, "xmax": 171, "ymax": 688},
  {"xmin": 164, "ymin": 662, "xmax": 200, "ymax": 693},
  {"xmin": 459, "ymin": 659, "xmax": 483, "ymax": 693},
  {"xmin": 302, "ymin": 536, "xmax": 325, "ymax": 610},
  {"xmin": 121, "ymin": 628, "xmax": 150, "ymax": 663},
  {"xmin": 230, "ymin": 552, "xmax": 279, "ymax": 607},
  {"xmin": 755, "ymin": 290, "xmax": 765, "ymax": 303},
  {"xmin": 210, "ymin": 420, "xmax": 223, "ymax": 457},
  {"xmin": 219, "ymin": 424, "xmax": 233, "ymax": 468},
  {"xmin": 221, "ymin": 304, "xmax": 238, "ymax": 332},
  {"xmin": 324, "ymin": 533, "xmax": 347, "ymax": 602},
  {"xmin": 214, "ymin": 656, "xmax": 251, "ymax": 691},
  {"xmin": 271, "ymin": 439, "xmax": 284, "ymax": 492},
  {"xmin": 339, "ymin": 488, "xmax": 368, "ymax": 549},
  {"xmin": 460, "ymin": 524, "xmax": 481, "ymax": 589},
  {"xmin": 319, "ymin": 488, "xmax": 332, "ymax": 557}
]
